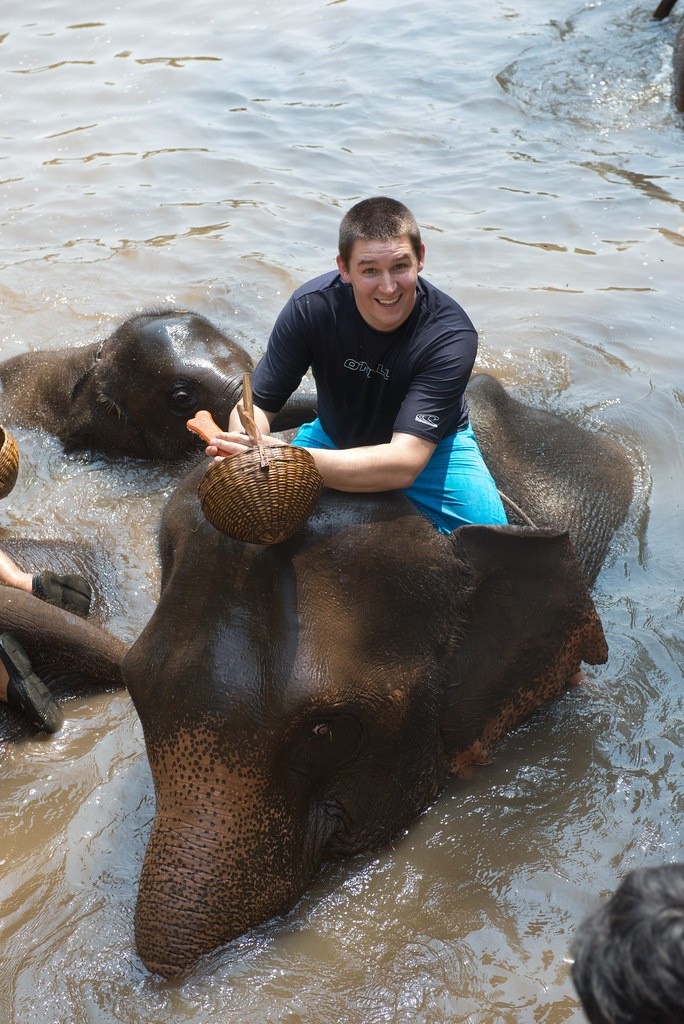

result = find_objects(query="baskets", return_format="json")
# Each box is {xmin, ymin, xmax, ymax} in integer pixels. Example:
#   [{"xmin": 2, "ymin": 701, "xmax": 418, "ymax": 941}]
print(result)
[
  {"xmin": 0, "ymin": 424, "xmax": 19, "ymax": 500},
  {"xmin": 197, "ymin": 374, "xmax": 325, "ymax": 545}
]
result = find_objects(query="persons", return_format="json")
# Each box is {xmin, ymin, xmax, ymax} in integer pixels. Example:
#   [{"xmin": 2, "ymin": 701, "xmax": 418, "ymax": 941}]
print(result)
[
  {"xmin": 205, "ymin": 196, "xmax": 510, "ymax": 536},
  {"xmin": 0, "ymin": 548, "xmax": 92, "ymax": 734}
]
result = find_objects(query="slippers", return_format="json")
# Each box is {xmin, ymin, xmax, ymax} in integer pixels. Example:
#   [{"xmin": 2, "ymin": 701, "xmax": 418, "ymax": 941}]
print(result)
[
  {"xmin": 32, "ymin": 571, "xmax": 91, "ymax": 615},
  {"xmin": 0, "ymin": 634, "xmax": 65, "ymax": 734}
]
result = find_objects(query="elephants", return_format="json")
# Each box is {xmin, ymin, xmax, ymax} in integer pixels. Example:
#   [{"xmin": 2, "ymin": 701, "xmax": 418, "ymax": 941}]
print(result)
[{"xmin": 0, "ymin": 311, "xmax": 633, "ymax": 972}]
